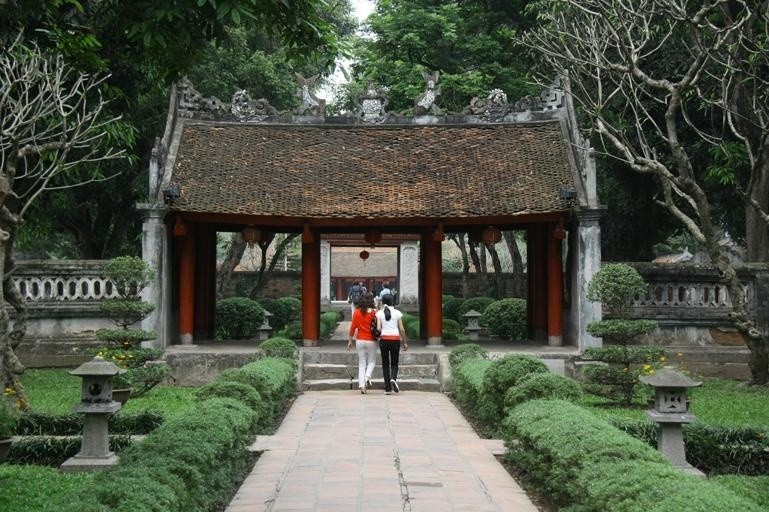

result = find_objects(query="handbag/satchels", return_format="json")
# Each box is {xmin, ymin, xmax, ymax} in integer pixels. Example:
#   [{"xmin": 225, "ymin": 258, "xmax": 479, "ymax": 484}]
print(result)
[{"xmin": 371, "ymin": 317, "xmax": 380, "ymax": 337}]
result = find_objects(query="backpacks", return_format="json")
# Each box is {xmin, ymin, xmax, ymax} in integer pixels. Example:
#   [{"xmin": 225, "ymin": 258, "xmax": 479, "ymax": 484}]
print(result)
[{"xmin": 352, "ymin": 286, "xmax": 361, "ymax": 305}]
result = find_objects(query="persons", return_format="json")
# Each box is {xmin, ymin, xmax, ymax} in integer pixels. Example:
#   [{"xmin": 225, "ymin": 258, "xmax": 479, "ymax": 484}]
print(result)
[
  {"xmin": 346, "ymin": 277, "xmax": 397, "ymax": 323},
  {"xmin": 346, "ymin": 292, "xmax": 379, "ymax": 394},
  {"xmin": 374, "ymin": 293, "xmax": 408, "ymax": 395}
]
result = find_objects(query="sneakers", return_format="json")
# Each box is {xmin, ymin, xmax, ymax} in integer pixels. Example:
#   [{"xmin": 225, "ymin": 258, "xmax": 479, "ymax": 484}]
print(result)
[
  {"xmin": 390, "ymin": 379, "xmax": 399, "ymax": 393},
  {"xmin": 384, "ymin": 390, "xmax": 391, "ymax": 395},
  {"xmin": 359, "ymin": 385, "xmax": 366, "ymax": 394},
  {"xmin": 366, "ymin": 378, "xmax": 372, "ymax": 389}
]
